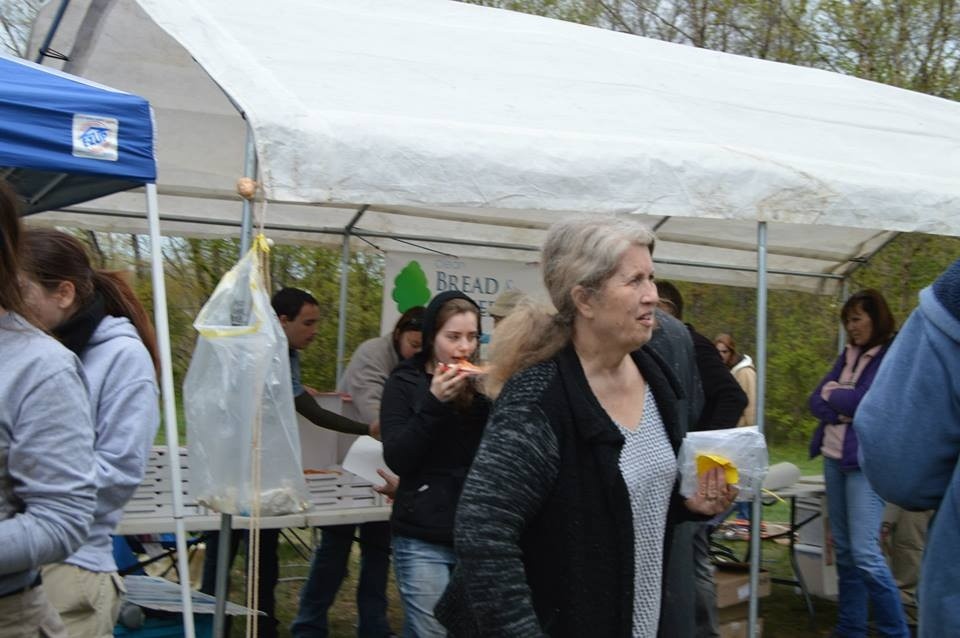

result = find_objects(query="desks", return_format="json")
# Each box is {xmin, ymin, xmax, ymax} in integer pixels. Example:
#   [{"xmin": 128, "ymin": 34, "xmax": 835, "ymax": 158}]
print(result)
[
  {"xmin": 114, "ymin": 504, "xmax": 393, "ymax": 638},
  {"xmin": 710, "ymin": 475, "xmax": 827, "ymax": 617}
]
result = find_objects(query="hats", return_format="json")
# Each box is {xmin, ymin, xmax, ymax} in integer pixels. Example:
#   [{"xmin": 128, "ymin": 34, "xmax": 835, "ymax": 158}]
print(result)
[{"xmin": 489, "ymin": 287, "xmax": 524, "ymax": 315}]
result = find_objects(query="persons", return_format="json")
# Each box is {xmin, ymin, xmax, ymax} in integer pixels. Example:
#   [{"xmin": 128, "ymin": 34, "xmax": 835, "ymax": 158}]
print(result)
[
  {"xmin": 853, "ymin": 257, "xmax": 960, "ymax": 638},
  {"xmin": 808, "ymin": 290, "xmax": 909, "ymax": 638},
  {"xmin": 200, "ymin": 287, "xmax": 380, "ymax": 638},
  {"xmin": 0, "ymin": 178, "xmax": 98, "ymax": 638},
  {"xmin": 648, "ymin": 308, "xmax": 704, "ymax": 638},
  {"xmin": 287, "ymin": 307, "xmax": 428, "ymax": 638},
  {"xmin": 379, "ymin": 291, "xmax": 494, "ymax": 638},
  {"xmin": 374, "ymin": 468, "xmax": 399, "ymax": 500},
  {"xmin": 490, "ymin": 286, "xmax": 523, "ymax": 326},
  {"xmin": 714, "ymin": 333, "xmax": 756, "ymax": 540},
  {"xmin": 14, "ymin": 228, "xmax": 161, "ymax": 638},
  {"xmin": 656, "ymin": 281, "xmax": 750, "ymax": 638},
  {"xmin": 432, "ymin": 210, "xmax": 739, "ymax": 638},
  {"xmin": 891, "ymin": 507, "xmax": 934, "ymax": 625}
]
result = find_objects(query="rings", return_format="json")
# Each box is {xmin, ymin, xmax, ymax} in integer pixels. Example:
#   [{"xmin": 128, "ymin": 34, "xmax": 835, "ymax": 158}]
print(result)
[{"xmin": 707, "ymin": 498, "xmax": 716, "ymax": 501}]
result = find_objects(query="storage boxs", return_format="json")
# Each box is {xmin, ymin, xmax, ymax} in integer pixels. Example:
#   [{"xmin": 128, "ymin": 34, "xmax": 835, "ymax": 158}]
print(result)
[
  {"xmin": 790, "ymin": 475, "xmax": 839, "ymax": 600},
  {"xmin": 122, "ymin": 391, "xmax": 384, "ymax": 515},
  {"xmin": 711, "ymin": 559, "xmax": 772, "ymax": 638}
]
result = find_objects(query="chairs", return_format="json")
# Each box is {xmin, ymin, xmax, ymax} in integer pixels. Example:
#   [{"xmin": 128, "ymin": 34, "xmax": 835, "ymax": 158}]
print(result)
[{"xmin": 115, "ymin": 536, "xmax": 199, "ymax": 584}]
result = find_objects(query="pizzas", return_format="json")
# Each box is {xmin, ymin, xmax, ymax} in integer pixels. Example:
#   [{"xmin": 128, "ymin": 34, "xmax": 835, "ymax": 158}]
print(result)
[{"xmin": 446, "ymin": 359, "xmax": 482, "ymax": 373}]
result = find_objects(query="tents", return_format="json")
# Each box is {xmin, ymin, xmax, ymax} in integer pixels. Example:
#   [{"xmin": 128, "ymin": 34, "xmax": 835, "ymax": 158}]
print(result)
[
  {"xmin": 27, "ymin": 0, "xmax": 960, "ymax": 638},
  {"xmin": 0, "ymin": 55, "xmax": 195, "ymax": 638}
]
